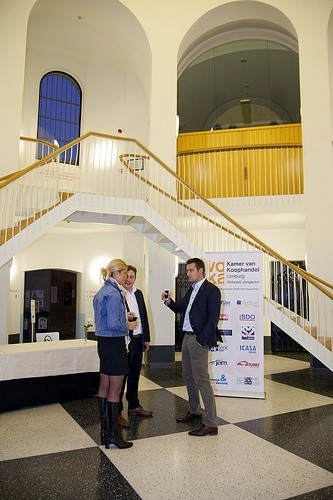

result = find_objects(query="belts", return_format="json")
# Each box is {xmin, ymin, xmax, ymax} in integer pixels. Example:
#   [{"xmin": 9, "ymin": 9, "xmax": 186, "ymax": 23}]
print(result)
[
  {"xmin": 132, "ymin": 335, "xmax": 142, "ymax": 338},
  {"xmin": 182, "ymin": 331, "xmax": 196, "ymax": 335}
]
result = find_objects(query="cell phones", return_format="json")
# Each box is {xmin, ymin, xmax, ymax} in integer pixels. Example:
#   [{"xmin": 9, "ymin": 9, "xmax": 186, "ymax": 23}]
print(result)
[{"xmin": 165, "ymin": 289, "xmax": 169, "ymax": 300}]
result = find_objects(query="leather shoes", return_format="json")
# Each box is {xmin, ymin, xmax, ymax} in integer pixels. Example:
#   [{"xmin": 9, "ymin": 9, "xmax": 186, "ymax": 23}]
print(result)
[
  {"xmin": 188, "ymin": 424, "xmax": 218, "ymax": 436},
  {"xmin": 176, "ymin": 411, "xmax": 202, "ymax": 422},
  {"xmin": 115, "ymin": 415, "xmax": 131, "ymax": 426},
  {"xmin": 128, "ymin": 407, "xmax": 153, "ymax": 416}
]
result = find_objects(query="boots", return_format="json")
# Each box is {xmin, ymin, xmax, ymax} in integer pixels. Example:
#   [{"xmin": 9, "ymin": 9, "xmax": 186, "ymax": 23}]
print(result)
[
  {"xmin": 98, "ymin": 396, "xmax": 106, "ymax": 445},
  {"xmin": 106, "ymin": 401, "xmax": 133, "ymax": 449}
]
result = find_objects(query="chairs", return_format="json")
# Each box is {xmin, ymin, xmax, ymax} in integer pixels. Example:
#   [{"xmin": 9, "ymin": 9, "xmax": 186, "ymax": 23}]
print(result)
[{"xmin": 35, "ymin": 331, "xmax": 60, "ymax": 343}]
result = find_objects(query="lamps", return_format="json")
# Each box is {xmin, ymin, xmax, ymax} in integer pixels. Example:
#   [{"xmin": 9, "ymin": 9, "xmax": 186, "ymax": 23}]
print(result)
[{"xmin": 239, "ymin": 57, "xmax": 253, "ymax": 124}]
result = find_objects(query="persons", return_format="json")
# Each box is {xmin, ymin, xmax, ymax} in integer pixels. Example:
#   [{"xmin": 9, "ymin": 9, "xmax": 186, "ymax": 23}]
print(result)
[
  {"xmin": 161, "ymin": 258, "xmax": 223, "ymax": 436},
  {"xmin": 116, "ymin": 263, "xmax": 153, "ymax": 426},
  {"xmin": 92, "ymin": 260, "xmax": 140, "ymax": 450}
]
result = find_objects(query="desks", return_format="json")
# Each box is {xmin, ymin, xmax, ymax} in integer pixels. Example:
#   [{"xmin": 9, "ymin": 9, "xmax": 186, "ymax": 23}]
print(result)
[{"xmin": 0, "ymin": 338, "xmax": 101, "ymax": 415}]
